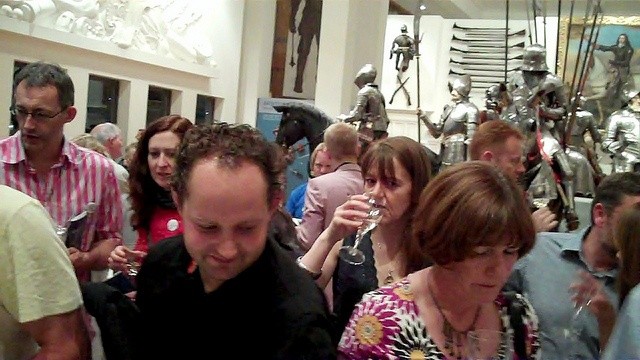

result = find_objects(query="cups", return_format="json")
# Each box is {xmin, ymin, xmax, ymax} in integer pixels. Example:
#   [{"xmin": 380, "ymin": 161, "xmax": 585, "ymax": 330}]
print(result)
[
  {"xmin": 465, "ymin": 328, "xmax": 508, "ymax": 359},
  {"xmin": 123, "ymin": 240, "xmax": 145, "ymax": 278}
]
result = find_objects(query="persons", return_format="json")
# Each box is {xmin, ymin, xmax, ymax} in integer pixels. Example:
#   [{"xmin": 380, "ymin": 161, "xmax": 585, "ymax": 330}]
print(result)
[
  {"xmin": 593, "ymin": 33, "xmax": 633, "ymax": 75},
  {"xmin": 69, "ymin": 132, "xmax": 108, "ymax": 157},
  {"xmin": 600, "ymin": 82, "xmax": 639, "ymax": 172},
  {"xmin": 416, "ymin": 74, "xmax": 480, "ymax": 173},
  {"xmin": 283, "ymin": 142, "xmax": 336, "ymax": 225},
  {"xmin": 90, "ymin": 121, "xmax": 130, "ymax": 183},
  {"xmin": 124, "ymin": 142, "xmax": 138, "ymax": 167},
  {"xmin": 288, "ymin": 0, "xmax": 322, "ymax": 94},
  {"xmin": 510, "ymin": 172, "xmax": 640, "ymax": 359},
  {"xmin": 107, "ymin": 114, "xmax": 213, "ymax": 300},
  {"xmin": 336, "ymin": 63, "xmax": 390, "ymax": 139},
  {"xmin": 132, "ymin": 120, "xmax": 334, "ymax": 359},
  {"xmin": 336, "ymin": 160, "xmax": 542, "ymax": 360},
  {"xmin": 563, "ymin": 96, "xmax": 605, "ymax": 181},
  {"xmin": 466, "ymin": 119, "xmax": 560, "ymax": 232},
  {"xmin": 294, "ymin": 135, "xmax": 433, "ymax": 337},
  {"xmin": 0, "ymin": 62, "xmax": 123, "ymax": 335},
  {"xmin": 390, "ymin": 24, "xmax": 417, "ymax": 71},
  {"xmin": 1, "ymin": 184, "xmax": 91, "ymax": 359},
  {"xmin": 502, "ymin": 44, "xmax": 580, "ymax": 230},
  {"xmin": 566, "ymin": 201, "xmax": 640, "ymax": 359},
  {"xmin": 294, "ymin": 121, "xmax": 365, "ymax": 251}
]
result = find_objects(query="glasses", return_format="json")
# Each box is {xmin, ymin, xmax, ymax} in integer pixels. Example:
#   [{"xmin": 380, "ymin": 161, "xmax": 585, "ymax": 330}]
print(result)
[{"xmin": 9, "ymin": 105, "xmax": 68, "ymax": 121}]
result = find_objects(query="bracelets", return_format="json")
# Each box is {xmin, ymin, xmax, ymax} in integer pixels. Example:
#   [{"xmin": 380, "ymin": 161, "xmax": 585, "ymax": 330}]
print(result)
[
  {"xmin": 296, "ymin": 255, "xmax": 322, "ymax": 280},
  {"xmin": 289, "ymin": 247, "xmax": 331, "ymax": 286}
]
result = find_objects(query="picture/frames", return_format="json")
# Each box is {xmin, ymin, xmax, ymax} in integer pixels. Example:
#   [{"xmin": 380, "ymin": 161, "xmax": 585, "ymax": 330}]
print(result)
[
  {"xmin": 556, "ymin": 15, "xmax": 640, "ymax": 99},
  {"xmin": 269, "ymin": 0, "xmax": 323, "ymax": 100}
]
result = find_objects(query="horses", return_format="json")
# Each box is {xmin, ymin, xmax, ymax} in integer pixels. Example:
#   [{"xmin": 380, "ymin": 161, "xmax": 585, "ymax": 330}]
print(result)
[
  {"xmin": 498, "ymin": 82, "xmax": 596, "ymax": 212},
  {"xmin": 271, "ymin": 100, "xmax": 373, "ymax": 180}
]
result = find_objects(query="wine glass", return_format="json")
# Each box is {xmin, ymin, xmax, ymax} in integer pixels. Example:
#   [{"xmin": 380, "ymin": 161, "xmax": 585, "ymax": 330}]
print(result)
[
  {"xmin": 337, "ymin": 188, "xmax": 388, "ymax": 266},
  {"xmin": 530, "ymin": 183, "xmax": 552, "ymax": 207}
]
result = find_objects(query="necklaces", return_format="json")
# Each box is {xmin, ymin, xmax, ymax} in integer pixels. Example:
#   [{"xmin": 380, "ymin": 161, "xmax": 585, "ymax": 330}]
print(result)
[
  {"xmin": 427, "ymin": 278, "xmax": 482, "ymax": 359},
  {"xmin": 377, "ymin": 228, "xmax": 406, "ymax": 285}
]
